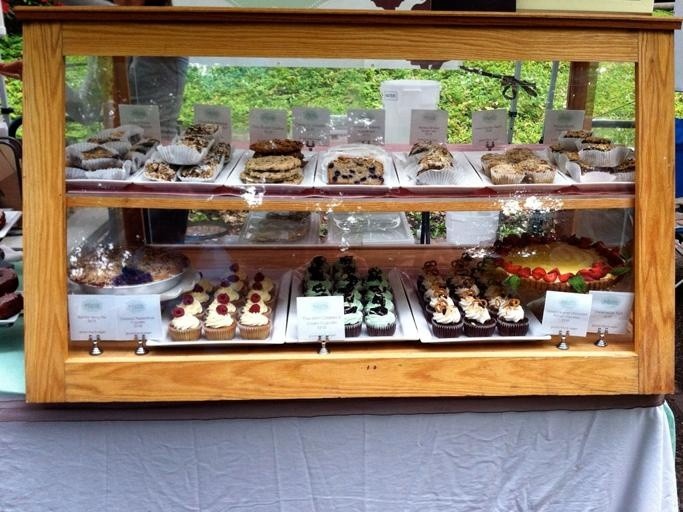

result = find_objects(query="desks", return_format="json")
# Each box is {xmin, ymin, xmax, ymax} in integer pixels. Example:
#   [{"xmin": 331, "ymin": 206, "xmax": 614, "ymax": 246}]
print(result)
[{"xmin": 0, "ymin": 395, "xmax": 679, "ymax": 512}]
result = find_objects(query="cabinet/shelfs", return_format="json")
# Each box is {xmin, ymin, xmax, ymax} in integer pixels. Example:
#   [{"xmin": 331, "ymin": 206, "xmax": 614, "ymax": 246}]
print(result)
[{"xmin": 14, "ymin": 4, "xmax": 683, "ymax": 404}]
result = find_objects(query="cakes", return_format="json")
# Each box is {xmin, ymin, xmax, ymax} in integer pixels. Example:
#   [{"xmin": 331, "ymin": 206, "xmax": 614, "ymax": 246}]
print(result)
[
  {"xmin": 0, "ymin": 264, "xmax": 27, "ymax": 325},
  {"xmin": 476, "ymin": 143, "xmax": 552, "ymax": 184},
  {"xmin": 143, "ymin": 124, "xmax": 234, "ymax": 182},
  {"xmin": 402, "ymin": 136, "xmax": 453, "ymax": 182},
  {"xmin": 411, "ymin": 256, "xmax": 529, "ymax": 337},
  {"xmin": 67, "ymin": 233, "xmax": 188, "ymax": 286},
  {"xmin": 164, "ymin": 259, "xmax": 274, "ymax": 342},
  {"xmin": 305, "ymin": 255, "xmax": 398, "ymax": 337},
  {"xmin": 472, "ymin": 233, "xmax": 622, "ymax": 336},
  {"xmin": 543, "ymin": 124, "xmax": 634, "ymax": 180},
  {"xmin": 64, "ymin": 120, "xmax": 161, "ymax": 180}
]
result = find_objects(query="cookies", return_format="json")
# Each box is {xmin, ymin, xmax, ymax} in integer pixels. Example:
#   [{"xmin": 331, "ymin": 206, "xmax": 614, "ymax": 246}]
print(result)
[
  {"xmin": 244, "ymin": 137, "xmax": 303, "ymax": 184},
  {"xmin": 327, "ymin": 155, "xmax": 387, "ymax": 186}
]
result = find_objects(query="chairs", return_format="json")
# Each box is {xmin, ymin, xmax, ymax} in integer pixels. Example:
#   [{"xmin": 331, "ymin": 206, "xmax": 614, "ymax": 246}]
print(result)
[{"xmin": 0, "ymin": 136, "xmax": 22, "ymax": 230}]
